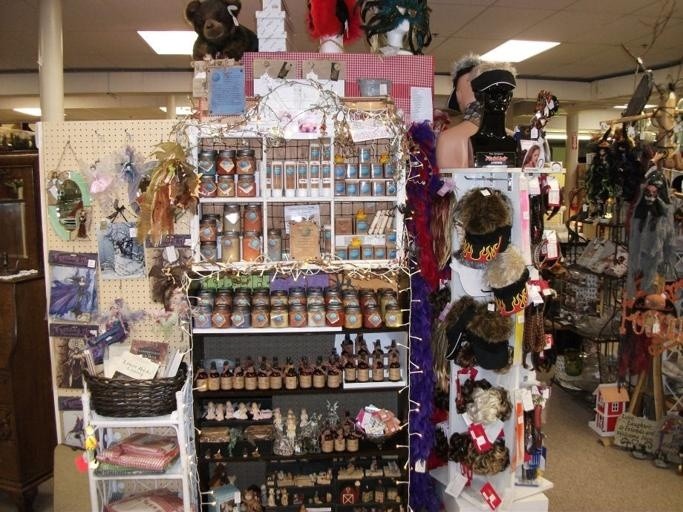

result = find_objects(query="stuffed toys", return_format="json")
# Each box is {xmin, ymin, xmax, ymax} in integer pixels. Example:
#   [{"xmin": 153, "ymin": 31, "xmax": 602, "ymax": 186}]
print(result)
[{"xmin": 181, "ymin": 0, "xmax": 257, "ymax": 61}]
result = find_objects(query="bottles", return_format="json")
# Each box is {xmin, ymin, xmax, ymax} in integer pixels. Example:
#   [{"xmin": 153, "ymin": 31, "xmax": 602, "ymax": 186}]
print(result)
[{"xmin": 196, "ymin": 332, "xmax": 400, "ymax": 505}]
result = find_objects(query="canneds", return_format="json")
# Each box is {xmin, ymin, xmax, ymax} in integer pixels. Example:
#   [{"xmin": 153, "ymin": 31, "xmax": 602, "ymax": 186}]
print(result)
[
  {"xmin": 194, "ymin": 287, "xmax": 401, "ymax": 330},
  {"xmin": 267, "ymin": 229, "xmax": 282, "ymax": 261},
  {"xmin": 198, "ymin": 177, "xmax": 217, "ymax": 198},
  {"xmin": 237, "ymin": 175, "xmax": 256, "ymax": 197},
  {"xmin": 216, "ymin": 149, "xmax": 236, "ymax": 175},
  {"xmin": 198, "ymin": 150, "xmax": 216, "ymax": 176},
  {"xmin": 199, "ymin": 204, "xmax": 241, "ymax": 262},
  {"xmin": 237, "ymin": 150, "xmax": 255, "ymax": 175},
  {"xmin": 241, "ymin": 231, "xmax": 261, "ymax": 261},
  {"xmin": 217, "ymin": 175, "xmax": 235, "ymax": 196},
  {"xmin": 243, "ymin": 204, "xmax": 262, "ymax": 233}
]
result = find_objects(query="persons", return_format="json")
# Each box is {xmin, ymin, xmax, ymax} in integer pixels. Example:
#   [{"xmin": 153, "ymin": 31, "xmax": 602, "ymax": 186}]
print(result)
[
  {"xmin": 467, "ymin": 62, "xmax": 521, "ymax": 168},
  {"xmin": 522, "ymin": 144, "xmax": 539, "ymax": 170},
  {"xmin": 353, "ymin": 0, "xmax": 431, "ymax": 56},
  {"xmin": 434, "ymin": 57, "xmax": 516, "ymax": 169},
  {"xmin": 304, "ymin": 0, "xmax": 363, "ymax": 55}
]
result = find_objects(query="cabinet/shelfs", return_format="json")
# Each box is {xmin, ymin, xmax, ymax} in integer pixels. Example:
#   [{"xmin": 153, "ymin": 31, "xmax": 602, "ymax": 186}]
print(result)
[
  {"xmin": 189, "ymin": 112, "xmax": 412, "ymax": 511},
  {"xmin": 81, "ymin": 376, "xmax": 194, "ymax": 512}
]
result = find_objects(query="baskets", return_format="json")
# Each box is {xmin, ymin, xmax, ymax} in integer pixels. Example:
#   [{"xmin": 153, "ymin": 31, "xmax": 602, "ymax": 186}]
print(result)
[{"xmin": 82, "ymin": 361, "xmax": 188, "ymax": 417}]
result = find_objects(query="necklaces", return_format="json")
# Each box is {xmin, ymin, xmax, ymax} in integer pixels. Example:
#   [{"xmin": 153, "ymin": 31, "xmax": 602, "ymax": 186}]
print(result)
[
  {"xmin": 386, "ymin": 45, "xmax": 404, "ymax": 55},
  {"xmin": 315, "ymin": 38, "xmax": 343, "ymax": 52}
]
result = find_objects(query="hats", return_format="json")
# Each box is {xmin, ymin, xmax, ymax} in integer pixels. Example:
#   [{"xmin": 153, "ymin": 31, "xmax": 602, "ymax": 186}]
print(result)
[
  {"xmin": 445, "ymin": 186, "xmax": 532, "ymax": 370},
  {"xmin": 446, "ymin": 56, "xmax": 488, "ymax": 111},
  {"xmin": 470, "ymin": 62, "xmax": 518, "ymax": 93}
]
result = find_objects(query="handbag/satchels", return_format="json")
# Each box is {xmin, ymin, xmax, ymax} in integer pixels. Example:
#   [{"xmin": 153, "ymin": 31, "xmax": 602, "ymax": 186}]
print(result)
[{"xmin": 524, "ymin": 312, "xmax": 546, "ymax": 353}]
